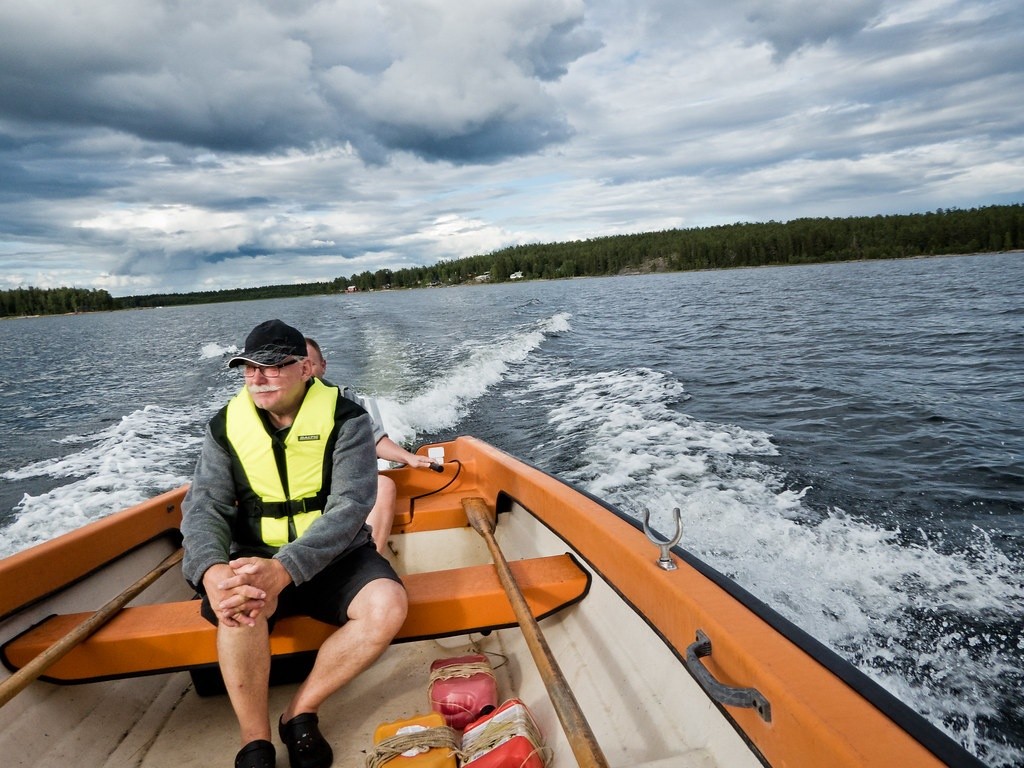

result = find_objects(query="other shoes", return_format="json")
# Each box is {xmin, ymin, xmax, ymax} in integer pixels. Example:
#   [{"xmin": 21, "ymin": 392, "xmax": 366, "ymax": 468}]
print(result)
[
  {"xmin": 234, "ymin": 739, "xmax": 276, "ymax": 768},
  {"xmin": 277, "ymin": 712, "xmax": 333, "ymax": 768}
]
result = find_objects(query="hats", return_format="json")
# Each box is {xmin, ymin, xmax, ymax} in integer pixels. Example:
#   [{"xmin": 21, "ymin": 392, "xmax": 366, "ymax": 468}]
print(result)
[{"xmin": 227, "ymin": 318, "xmax": 308, "ymax": 368}]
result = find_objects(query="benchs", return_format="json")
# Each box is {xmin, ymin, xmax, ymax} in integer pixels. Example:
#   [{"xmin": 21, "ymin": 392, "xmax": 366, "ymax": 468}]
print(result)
[
  {"xmin": 177, "ymin": 488, "xmax": 494, "ymax": 577},
  {"xmin": 0, "ymin": 553, "xmax": 591, "ymax": 686}
]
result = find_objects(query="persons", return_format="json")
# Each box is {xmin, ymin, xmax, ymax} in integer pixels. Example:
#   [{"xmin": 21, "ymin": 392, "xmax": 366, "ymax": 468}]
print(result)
[
  {"xmin": 179, "ymin": 318, "xmax": 409, "ymax": 768},
  {"xmin": 305, "ymin": 338, "xmax": 438, "ymax": 554}
]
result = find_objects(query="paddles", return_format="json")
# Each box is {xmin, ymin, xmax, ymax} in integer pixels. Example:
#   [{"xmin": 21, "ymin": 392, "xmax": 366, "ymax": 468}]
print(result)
[
  {"xmin": 458, "ymin": 493, "xmax": 612, "ymax": 768},
  {"xmin": 0, "ymin": 545, "xmax": 188, "ymax": 711}
]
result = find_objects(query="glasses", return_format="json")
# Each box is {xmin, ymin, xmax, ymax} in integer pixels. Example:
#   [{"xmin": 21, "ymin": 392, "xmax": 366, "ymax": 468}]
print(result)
[{"xmin": 236, "ymin": 359, "xmax": 298, "ymax": 378}]
